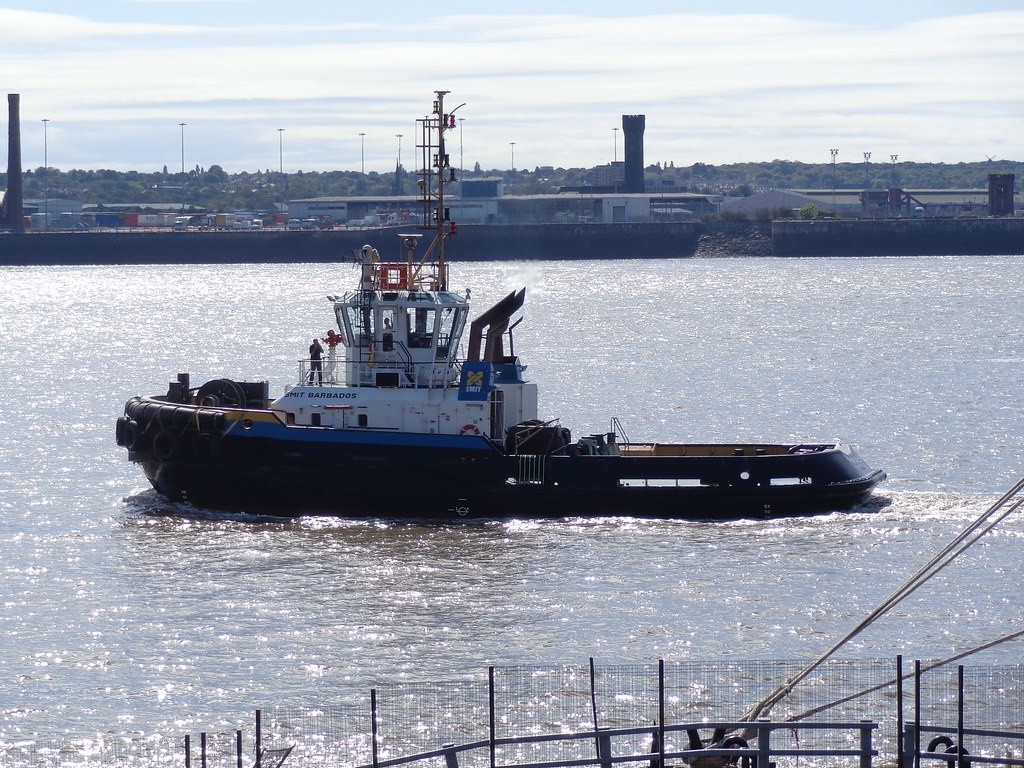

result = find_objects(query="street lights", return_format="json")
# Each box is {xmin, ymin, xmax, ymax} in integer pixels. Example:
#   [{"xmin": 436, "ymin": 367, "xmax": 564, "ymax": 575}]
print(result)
[
  {"xmin": 179, "ymin": 123, "xmax": 186, "ymax": 175},
  {"xmin": 359, "ymin": 133, "xmax": 366, "ymax": 174},
  {"xmin": 612, "ymin": 128, "xmax": 618, "ymax": 163},
  {"xmin": 41, "ymin": 119, "xmax": 50, "ymax": 232},
  {"xmin": 396, "ymin": 133, "xmax": 403, "ymax": 167},
  {"xmin": 510, "ymin": 142, "xmax": 515, "ymax": 169},
  {"xmin": 830, "ymin": 148, "xmax": 839, "ymax": 214},
  {"xmin": 863, "ymin": 152, "xmax": 871, "ymax": 217},
  {"xmin": 276, "ymin": 128, "xmax": 285, "ymax": 174},
  {"xmin": 458, "ymin": 118, "xmax": 466, "ymax": 171},
  {"xmin": 889, "ymin": 155, "xmax": 899, "ymax": 187}
]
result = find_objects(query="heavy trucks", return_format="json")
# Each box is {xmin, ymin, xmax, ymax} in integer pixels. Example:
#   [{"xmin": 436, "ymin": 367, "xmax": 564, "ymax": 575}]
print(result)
[{"xmin": 386, "ymin": 212, "xmax": 428, "ymax": 224}]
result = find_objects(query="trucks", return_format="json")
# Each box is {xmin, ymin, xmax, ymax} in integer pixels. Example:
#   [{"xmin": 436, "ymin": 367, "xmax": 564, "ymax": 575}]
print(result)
[
  {"xmin": 174, "ymin": 216, "xmax": 192, "ymax": 231},
  {"xmin": 252, "ymin": 219, "xmax": 263, "ymax": 230},
  {"xmin": 198, "ymin": 215, "xmax": 215, "ymax": 231},
  {"xmin": 345, "ymin": 219, "xmax": 365, "ymax": 227}
]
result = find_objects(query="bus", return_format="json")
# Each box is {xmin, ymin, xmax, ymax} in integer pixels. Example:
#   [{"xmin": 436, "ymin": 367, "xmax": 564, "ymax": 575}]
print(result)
[
  {"xmin": 288, "ymin": 219, "xmax": 301, "ymax": 230},
  {"xmin": 114, "ymin": 92, "xmax": 888, "ymax": 527},
  {"xmin": 302, "ymin": 219, "xmax": 316, "ymax": 230}
]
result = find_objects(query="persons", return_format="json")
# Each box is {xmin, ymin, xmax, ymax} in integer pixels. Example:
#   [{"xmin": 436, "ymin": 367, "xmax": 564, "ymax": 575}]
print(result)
[
  {"xmin": 309, "ymin": 338, "xmax": 325, "ymax": 386},
  {"xmin": 384, "ymin": 318, "xmax": 392, "ymax": 329}
]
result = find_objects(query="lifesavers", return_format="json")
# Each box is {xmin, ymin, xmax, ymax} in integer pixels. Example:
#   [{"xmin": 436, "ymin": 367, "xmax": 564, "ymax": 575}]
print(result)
[
  {"xmin": 192, "ymin": 432, "xmax": 220, "ymax": 464},
  {"xmin": 115, "ymin": 416, "xmax": 129, "ymax": 446},
  {"xmin": 459, "ymin": 424, "xmax": 480, "ymax": 435},
  {"xmin": 153, "ymin": 430, "xmax": 183, "ymax": 463},
  {"xmin": 123, "ymin": 420, "xmax": 142, "ymax": 452}
]
